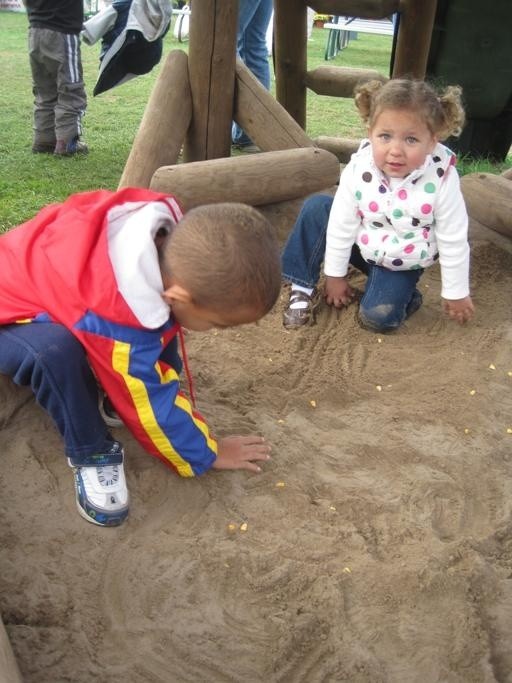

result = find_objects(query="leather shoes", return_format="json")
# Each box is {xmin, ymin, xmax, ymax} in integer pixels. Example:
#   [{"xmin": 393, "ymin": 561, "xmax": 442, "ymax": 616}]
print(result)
[{"xmin": 283, "ymin": 288, "xmax": 321, "ymax": 331}]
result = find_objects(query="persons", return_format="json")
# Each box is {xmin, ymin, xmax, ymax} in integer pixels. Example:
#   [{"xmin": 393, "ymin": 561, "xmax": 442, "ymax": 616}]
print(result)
[
  {"xmin": 281, "ymin": 75, "xmax": 476, "ymax": 333},
  {"xmin": 231, "ymin": 0, "xmax": 273, "ymax": 146},
  {"xmin": 0, "ymin": 187, "xmax": 281, "ymax": 527},
  {"xmin": 23, "ymin": 0, "xmax": 88, "ymax": 157}
]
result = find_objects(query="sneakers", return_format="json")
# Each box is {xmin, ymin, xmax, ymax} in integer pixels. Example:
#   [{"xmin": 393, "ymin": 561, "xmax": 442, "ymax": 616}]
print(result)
[
  {"xmin": 68, "ymin": 442, "xmax": 129, "ymax": 527},
  {"xmin": 233, "ymin": 143, "xmax": 260, "ymax": 151},
  {"xmin": 98, "ymin": 392, "xmax": 124, "ymax": 427},
  {"xmin": 34, "ymin": 142, "xmax": 88, "ymax": 159}
]
